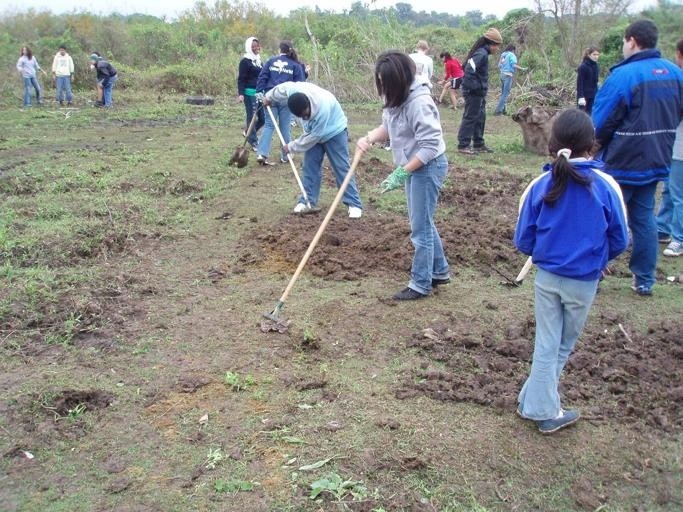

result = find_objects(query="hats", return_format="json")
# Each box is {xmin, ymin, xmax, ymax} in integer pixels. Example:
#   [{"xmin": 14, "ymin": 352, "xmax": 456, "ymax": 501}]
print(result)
[
  {"xmin": 88, "ymin": 54, "xmax": 98, "ymax": 61},
  {"xmin": 483, "ymin": 27, "xmax": 503, "ymax": 44}
]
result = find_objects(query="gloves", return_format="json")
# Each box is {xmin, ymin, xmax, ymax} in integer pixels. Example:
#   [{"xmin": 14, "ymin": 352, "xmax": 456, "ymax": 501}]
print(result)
[
  {"xmin": 522, "ymin": 67, "xmax": 528, "ymax": 72},
  {"xmin": 577, "ymin": 98, "xmax": 586, "ymax": 106},
  {"xmin": 380, "ymin": 166, "xmax": 412, "ymax": 194}
]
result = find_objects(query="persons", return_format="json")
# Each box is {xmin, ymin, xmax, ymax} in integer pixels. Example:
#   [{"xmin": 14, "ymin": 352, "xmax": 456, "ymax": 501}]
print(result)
[
  {"xmin": 262, "ymin": 81, "xmax": 362, "ymax": 219},
  {"xmin": 457, "ymin": 27, "xmax": 503, "ymax": 155},
  {"xmin": 16, "ymin": 45, "xmax": 47, "ymax": 106},
  {"xmin": 435, "ymin": 51, "xmax": 465, "ymax": 109},
  {"xmin": 236, "ymin": 36, "xmax": 264, "ymax": 152},
  {"xmin": 255, "ymin": 40, "xmax": 310, "ymax": 165},
  {"xmin": 513, "ymin": 107, "xmax": 630, "ymax": 435},
  {"xmin": 576, "ymin": 44, "xmax": 600, "ymax": 117},
  {"xmin": 589, "ymin": 21, "xmax": 683, "ymax": 296},
  {"xmin": 88, "ymin": 54, "xmax": 118, "ymax": 108},
  {"xmin": 92, "ymin": 52, "xmax": 103, "ymax": 106},
  {"xmin": 493, "ymin": 43, "xmax": 528, "ymax": 116},
  {"xmin": 357, "ymin": 50, "xmax": 451, "ymax": 300},
  {"xmin": 51, "ymin": 45, "xmax": 75, "ymax": 106},
  {"xmin": 407, "ymin": 40, "xmax": 433, "ymax": 80},
  {"xmin": 654, "ymin": 38, "xmax": 683, "ymax": 257}
]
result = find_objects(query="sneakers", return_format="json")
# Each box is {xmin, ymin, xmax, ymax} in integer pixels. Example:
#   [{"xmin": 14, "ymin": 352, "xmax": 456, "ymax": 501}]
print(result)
[
  {"xmin": 473, "ymin": 146, "xmax": 492, "ymax": 153},
  {"xmin": 537, "ymin": 407, "xmax": 581, "ymax": 433},
  {"xmin": 458, "ymin": 146, "xmax": 476, "ymax": 154},
  {"xmin": 293, "ymin": 203, "xmax": 306, "ymax": 213},
  {"xmin": 243, "ymin": 130, "xmax": 294, "ymax": 165},
  {"xmin": 657, "ymin": 231, "xmax": 671, "ymax": 243},
  {"xmin": 24, "ymin": 101, "xmax": 72, "ymax": 107},
  {"xmin": 662, "ymin": 240, "xmax": 682, "ymax": 257},
  {"xmin": 432, "ymin": 279, "xmax": 450, "ymax": 285},
  {"xmin": 394, "ymin": 287, "xmax": 426, "ymax": 300},
  {"xmin": 347, "ymin": 206, "xmax": 362, "ymax": 218},
  {"xmin": 632, "ymin": 274, "xmax": 653, "ymax": 295}
]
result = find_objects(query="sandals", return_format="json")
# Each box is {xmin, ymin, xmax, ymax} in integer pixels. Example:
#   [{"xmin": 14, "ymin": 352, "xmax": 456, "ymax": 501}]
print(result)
[{"xmin": 433, "ymin": 96, "xmax": 442, "ymax": 104}]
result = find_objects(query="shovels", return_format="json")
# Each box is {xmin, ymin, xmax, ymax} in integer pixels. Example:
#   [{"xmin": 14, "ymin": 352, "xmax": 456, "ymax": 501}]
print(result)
[{"xmin": 229, "ymin": 98, "xmax": 264, "ymax": 169}]
